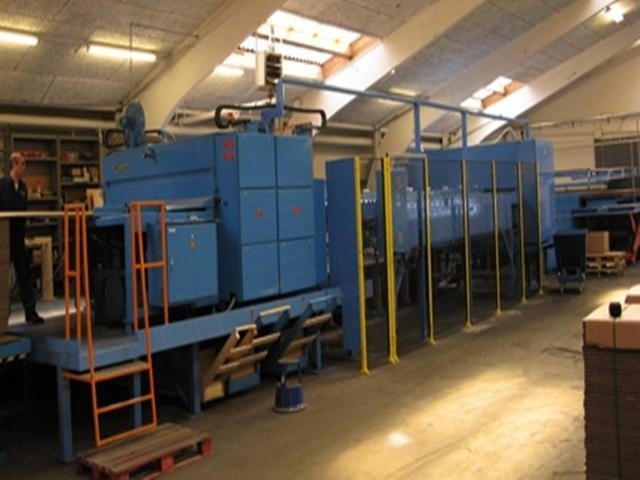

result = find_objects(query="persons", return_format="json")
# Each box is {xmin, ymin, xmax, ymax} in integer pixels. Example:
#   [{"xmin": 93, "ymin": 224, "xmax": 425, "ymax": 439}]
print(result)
[{"xmin": 0, "ymin": 151, "xmax": 45, "ymax": 324}]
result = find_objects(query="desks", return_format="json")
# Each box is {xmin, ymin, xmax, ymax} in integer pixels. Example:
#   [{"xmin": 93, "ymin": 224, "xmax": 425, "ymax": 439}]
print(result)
[{"xmin": 585, "ymin": 251, "xmax": 626, "ymax": 279}]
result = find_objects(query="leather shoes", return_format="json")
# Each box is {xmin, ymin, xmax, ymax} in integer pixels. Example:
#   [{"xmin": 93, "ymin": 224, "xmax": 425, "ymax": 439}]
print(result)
[{"xmin": 24, "ymin": 314, "xmax": 45, "ymax": 324}]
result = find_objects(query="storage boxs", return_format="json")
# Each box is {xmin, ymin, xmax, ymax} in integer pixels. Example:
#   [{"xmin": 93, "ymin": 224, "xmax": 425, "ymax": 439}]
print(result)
[
  {"xmin": 587, "ymin": 230, "xmax": 609, "ymax": 253},
  {"xmin": 583, "ymin": 283, "xmax": 640, "ymax": 480}
]
